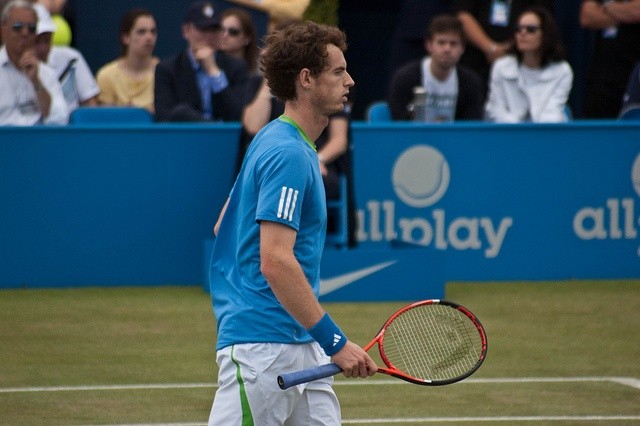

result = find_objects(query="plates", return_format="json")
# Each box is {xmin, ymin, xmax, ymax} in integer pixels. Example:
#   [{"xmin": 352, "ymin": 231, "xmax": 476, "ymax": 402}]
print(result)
[{"xmin": 407, "ymin": 85, "xmax": 427, "ymax": 122}]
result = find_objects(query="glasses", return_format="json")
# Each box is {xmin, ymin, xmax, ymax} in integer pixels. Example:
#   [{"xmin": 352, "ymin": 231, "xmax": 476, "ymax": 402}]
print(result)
[
  {"xmin": 514, "ymin": 27, "xmax": 543, "ymax": 33},
  {"xmin": 6, "ymin": 22, "xmax": 36, "ymax": 34},
  {"xmin": 221, "ymin": 25, "xmax": 242, "ymax": 36}
]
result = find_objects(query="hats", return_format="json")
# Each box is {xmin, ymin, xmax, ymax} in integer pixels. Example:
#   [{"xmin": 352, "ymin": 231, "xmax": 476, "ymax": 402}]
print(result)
[
  {"xmin": 35, "ymin": 4, "xmax": 57, "ymax": 35},
  {"xmin": 183, "ymin": 1, "xmax": 220, "ymax": 28}
]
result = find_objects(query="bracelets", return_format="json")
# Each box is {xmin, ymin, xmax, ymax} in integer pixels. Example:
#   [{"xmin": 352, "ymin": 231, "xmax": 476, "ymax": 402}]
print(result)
[{"xmin": 307, "ymin": 312, "xmax": 347, "ymax": 356}]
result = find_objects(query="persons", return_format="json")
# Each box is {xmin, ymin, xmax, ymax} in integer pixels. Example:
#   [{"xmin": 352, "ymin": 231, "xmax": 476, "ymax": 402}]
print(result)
[
  {"xmin": 232, "ymin": 44, "xmax": 357, "ymax": 179},
  {"xmin": 207, "ymin": 20, "xmax": 378, "ymax": 426},
  {"xmin": 154, "ymin": 0, "xmax": 263, "ymax": 122},
  {"xmin": 38, "ymin": 0, "xmax": 71, "ymax": 48},
  {"xmin": 0, "ymin": 1, "xmax": 69, "ymax": 126},
  {"xmin": 570, "ymin": 0, "xmax": 639, "ymax": 120},
  {"xmin": 387, "ymin": 14, "xmax": 488, "ymax": 120},
  {"xmin": 32, "ymin": 3, "xmax": 101, "ymax": 117},
  {"xmin": 458, "ymin": 1, "xmax": 517, "ymax": 85},
  {"xmin": 483, "ymin": 8, "xmax": 574, "ymax": 124},
  {"xmin": 95, "ymin": 7, "xmax": 162, "ymax": 115},
  {"xmin": 219, "ymin": 9, "xmax": 257, "ymax": 71}
]
